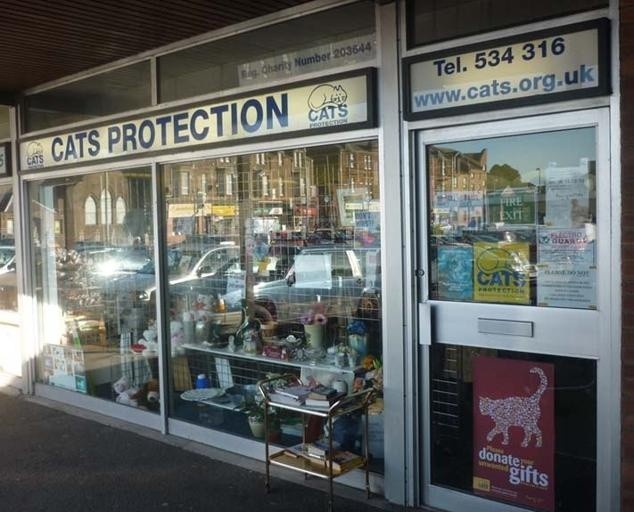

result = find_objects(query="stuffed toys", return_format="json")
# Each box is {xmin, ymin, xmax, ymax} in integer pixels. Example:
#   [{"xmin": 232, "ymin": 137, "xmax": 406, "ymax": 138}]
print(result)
[
  {"xmin": 113, "ymin": 376, "xmax": 160, "ymax": 414},
  {"xmin": 138, "ymin": 321, "xmax": 185, "ymax": 358}
]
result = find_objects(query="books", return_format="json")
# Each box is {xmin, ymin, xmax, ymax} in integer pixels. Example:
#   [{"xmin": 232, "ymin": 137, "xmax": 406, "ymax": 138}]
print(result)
[
  {"xmin": 283, "ymin": 437, "xmax": 362, "ymax": 472},
  {"xmin": 270, "ymin": 384, "xmax": 346, "ymax": 412}
]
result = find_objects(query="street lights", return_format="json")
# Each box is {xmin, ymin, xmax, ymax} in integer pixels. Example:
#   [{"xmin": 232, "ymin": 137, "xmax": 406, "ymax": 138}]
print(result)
[{"xmin": 535, "ymin": 167, "xmax": 542, "ymax": 192}]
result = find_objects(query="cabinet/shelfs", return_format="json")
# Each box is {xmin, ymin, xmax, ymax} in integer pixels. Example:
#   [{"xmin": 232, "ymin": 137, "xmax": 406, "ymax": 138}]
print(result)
[{"xmin": 183, "ymin": 342, "xmax": 356, "ymax": 438}]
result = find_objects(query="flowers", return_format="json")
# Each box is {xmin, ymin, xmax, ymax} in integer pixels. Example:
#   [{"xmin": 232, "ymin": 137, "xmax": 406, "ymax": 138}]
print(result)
[
  {"xmin": 299, "ymin": 309, "xmax": 327, "ymax": 326},
  {"xmin": 346, "ymin": 319, "xmax": 367, "ymax": 335}
]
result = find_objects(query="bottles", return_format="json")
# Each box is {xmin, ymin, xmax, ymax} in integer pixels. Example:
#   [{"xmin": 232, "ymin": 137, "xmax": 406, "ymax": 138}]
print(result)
[{"xmin": 196, "ymin": 374, "xmax": 209, "ymax": 388}]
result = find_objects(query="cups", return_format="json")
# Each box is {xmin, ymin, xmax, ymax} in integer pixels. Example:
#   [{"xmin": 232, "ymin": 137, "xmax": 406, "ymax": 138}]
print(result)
[
  {"xmin": 260, "ymin": 322, "xmax": 277, "ymax": 341},
  {"xmin": 334, "ymin": 351, "xmax": 345, "ymax": 366}
]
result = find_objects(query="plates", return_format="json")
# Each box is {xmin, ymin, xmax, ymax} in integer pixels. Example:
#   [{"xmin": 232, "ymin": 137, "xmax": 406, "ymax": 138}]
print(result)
[{"xmin": 180, "ymin": 388, "xmax": 217, "ymax": 401}]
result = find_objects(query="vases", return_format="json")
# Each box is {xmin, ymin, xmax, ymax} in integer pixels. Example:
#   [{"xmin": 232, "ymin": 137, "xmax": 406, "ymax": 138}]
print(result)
[
  {"xmin": 304, "ymin": 323, "xmax": 324, "ymax": 351},
  {"xmin": 349, "ymin": 335, "xmax": 370, "ymax": 359}
]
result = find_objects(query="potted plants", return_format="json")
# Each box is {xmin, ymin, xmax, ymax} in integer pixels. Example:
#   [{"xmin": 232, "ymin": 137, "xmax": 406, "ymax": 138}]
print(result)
[{"xmin": 232, "ymin": 393, "xmax": 302, "ymax": 440}]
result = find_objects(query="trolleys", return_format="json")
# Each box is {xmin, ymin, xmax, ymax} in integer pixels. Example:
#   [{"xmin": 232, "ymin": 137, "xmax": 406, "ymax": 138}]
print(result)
[{"xmin": 251, "ymin": 373, "xmax": 380, "ymax": 512}]
